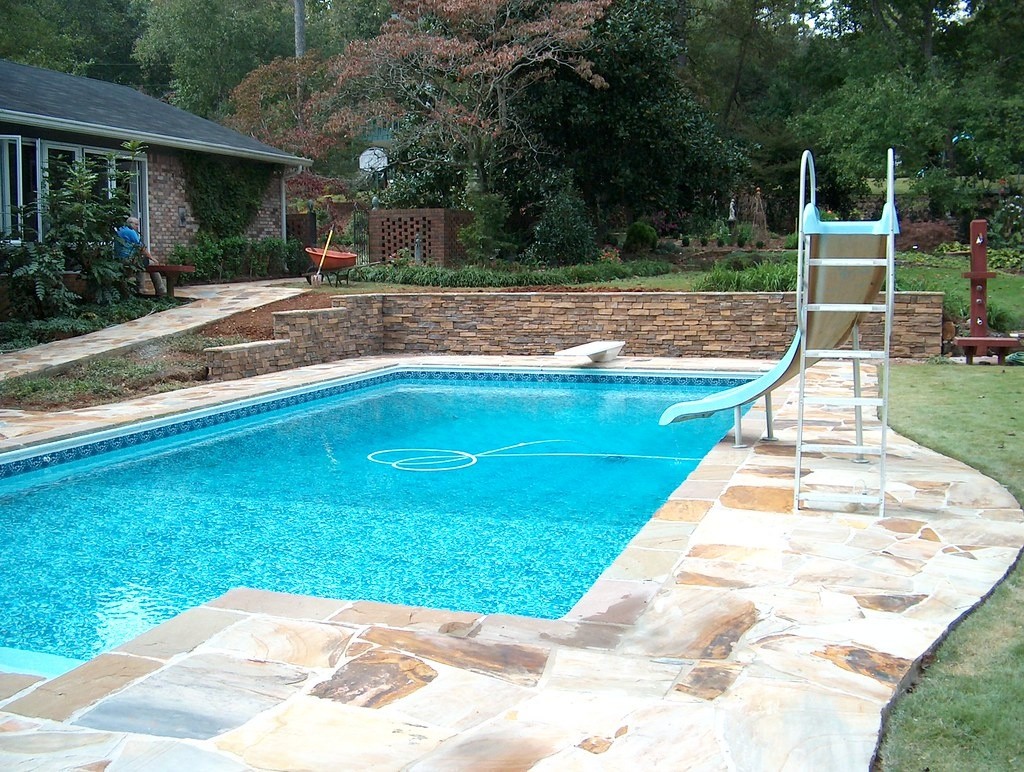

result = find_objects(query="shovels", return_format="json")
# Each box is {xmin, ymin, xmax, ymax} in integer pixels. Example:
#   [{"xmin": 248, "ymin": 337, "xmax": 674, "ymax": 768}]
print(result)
[{"xmin": 311, "ymin": 224, "xmax": 335, "ymax": 288}]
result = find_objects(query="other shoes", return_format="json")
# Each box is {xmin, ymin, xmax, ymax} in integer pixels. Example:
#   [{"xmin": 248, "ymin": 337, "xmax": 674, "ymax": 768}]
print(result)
[{"xmin": 156, "ymin": 290, "xmax": 168, "ymax": 296}]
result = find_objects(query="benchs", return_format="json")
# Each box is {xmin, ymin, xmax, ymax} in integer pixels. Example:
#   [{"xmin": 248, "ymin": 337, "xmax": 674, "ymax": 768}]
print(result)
[
  {"xmin": 145, "ymin": 265, "xmax": 195, "ymax": 299},
  {"xmin": 954, "ymin": 337, "xmax": 1018, "ymax": 365}
]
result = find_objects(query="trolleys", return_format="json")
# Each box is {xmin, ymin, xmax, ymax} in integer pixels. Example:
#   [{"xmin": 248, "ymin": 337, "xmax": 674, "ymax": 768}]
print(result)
[{"xmin": 303, "ymin": 247, "xmax": 386, "ymax": 289}]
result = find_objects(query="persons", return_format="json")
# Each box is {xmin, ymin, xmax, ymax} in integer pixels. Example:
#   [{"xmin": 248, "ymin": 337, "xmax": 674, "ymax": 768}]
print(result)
[{"xmin": 115, "ymin": 217, "xmax": 159, "ymax": 293}]
